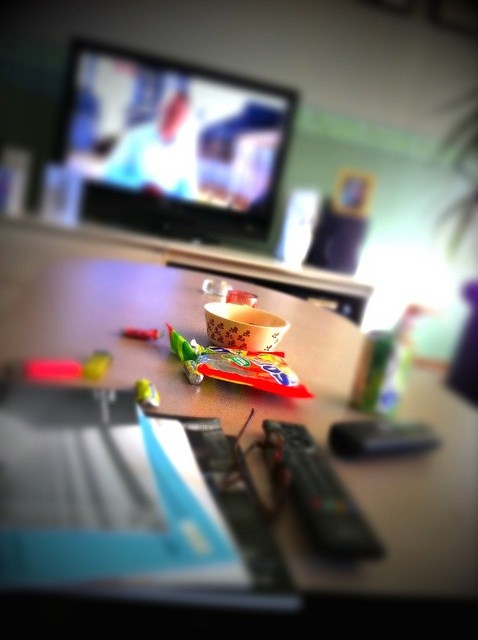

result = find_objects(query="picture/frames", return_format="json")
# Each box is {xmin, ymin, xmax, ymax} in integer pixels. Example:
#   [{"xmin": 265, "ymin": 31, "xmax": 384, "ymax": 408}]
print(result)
[{"xmin": 334, "ymin": 168, "xmax": 380, "ymax": 217}]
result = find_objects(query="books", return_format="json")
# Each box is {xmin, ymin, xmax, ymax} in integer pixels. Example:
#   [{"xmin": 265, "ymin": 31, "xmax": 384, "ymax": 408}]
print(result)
[{"xmin": 2, "ymin": 372, "xmax": 299, "ymax": 610}]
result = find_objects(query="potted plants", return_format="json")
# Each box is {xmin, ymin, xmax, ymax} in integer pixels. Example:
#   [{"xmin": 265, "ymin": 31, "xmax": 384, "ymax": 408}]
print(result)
[{"xmin": 429, "ymin": 84, "xmax": 478, "ymax": 406}]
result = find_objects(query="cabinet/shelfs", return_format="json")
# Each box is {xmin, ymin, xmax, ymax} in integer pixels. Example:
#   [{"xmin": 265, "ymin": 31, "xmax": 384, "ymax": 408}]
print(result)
[{"xmin": 0, "ymin": 216, "xmax": 374, "ymax": 324}]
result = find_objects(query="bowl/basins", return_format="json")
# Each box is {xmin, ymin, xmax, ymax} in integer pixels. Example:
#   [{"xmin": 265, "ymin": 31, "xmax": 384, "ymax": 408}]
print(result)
[{"xmin": 203, "ymin": 302, "xmax": 292, "ymax": 353}]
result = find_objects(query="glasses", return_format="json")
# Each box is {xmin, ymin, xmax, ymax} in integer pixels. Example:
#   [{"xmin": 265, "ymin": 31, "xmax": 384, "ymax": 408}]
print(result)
[{"xmin": 224, "ymin": 408, "xmax": 290, "ymax": 522}]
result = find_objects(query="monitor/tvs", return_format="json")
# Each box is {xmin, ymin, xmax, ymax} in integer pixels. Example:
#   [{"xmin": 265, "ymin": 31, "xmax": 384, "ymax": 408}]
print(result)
[{"xmin": 47, "ymin": 32, "xmax": 299, "ymax": 245}]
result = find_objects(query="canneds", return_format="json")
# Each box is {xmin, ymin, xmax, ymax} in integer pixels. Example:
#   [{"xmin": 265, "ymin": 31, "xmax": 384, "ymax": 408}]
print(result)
[{"xmin": 349, "ymin": 330, "xmax": 412, "ymax": 414}]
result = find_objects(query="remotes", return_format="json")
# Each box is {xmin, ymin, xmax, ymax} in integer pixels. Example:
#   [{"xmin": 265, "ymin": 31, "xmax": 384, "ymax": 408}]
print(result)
[
  {"xmin": 332, "ymin": 418, "xmax": 443, "ymax": 459},
  {"xmin": 261, "ymin": 418, "xmax": 385, "ymax": 557}
]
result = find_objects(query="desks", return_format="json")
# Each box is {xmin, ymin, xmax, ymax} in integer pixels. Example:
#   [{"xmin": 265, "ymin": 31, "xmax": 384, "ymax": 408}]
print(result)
[{"xmin": 1, "ymin": 259, "xmax": 478, "ymax": 601}]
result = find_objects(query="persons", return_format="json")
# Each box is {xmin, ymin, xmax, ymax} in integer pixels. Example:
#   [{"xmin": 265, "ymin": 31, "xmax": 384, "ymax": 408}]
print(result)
[{"xmin": 98, "ymin": 92, "xmax": 198, "ymax": 202}]
